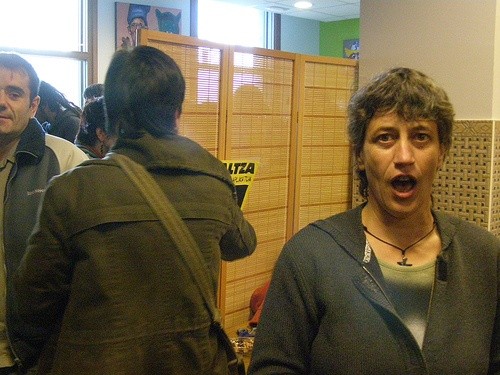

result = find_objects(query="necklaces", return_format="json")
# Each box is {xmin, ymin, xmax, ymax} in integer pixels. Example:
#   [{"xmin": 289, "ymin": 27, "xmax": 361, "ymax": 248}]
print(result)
[{"xmin": 360, "ymin": 222, "xmax": 438, "ymax": 269}]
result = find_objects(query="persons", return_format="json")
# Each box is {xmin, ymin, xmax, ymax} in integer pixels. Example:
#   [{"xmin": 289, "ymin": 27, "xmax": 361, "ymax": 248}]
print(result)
[
  {"xmin": 0, "ymin": 53, "xmax": 92, "ymax": 374},
  {"xmin": 82, "ymin": 82, "xmax": 107, "ymax": 107},
  {"xmin": 7, "ymin": 44, "xmax": 259, "ymax": 375},
  {"xmin": 32, "ymin": 77, "xmax": 84, "ymax": 150},
  {"xmin": 73, "ymin": 95, "xmax": 118, "ymax": 163},
  {"xmin": 248, "ymin": 66, "xmax": 500, "ymax": 375}
]
